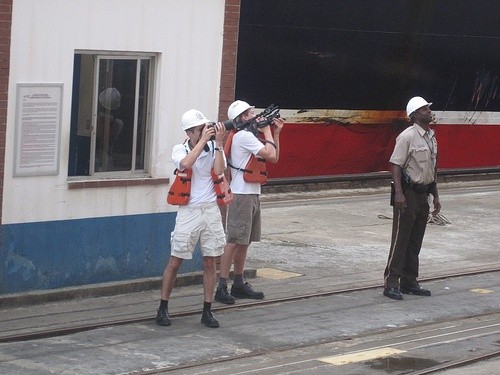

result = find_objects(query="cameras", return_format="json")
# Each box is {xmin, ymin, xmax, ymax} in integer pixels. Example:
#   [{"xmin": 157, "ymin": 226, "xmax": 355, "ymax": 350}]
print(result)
[{"xmin": 208, "ymin": 119, "xmax": 237, "ymax": 140}]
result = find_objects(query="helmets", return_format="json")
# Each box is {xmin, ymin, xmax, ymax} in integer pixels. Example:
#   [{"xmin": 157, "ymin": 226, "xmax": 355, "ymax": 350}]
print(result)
[
  {"xmin": 181, "ymin": 109, "xmax": 209, "ymax": 131},
  {"xmin": 407, "ymin": 96, "xmax": 433, "ymax": 118},
  {"xmin": 227, "ymin": 100, "xmax": 255, "ymax": 120}
]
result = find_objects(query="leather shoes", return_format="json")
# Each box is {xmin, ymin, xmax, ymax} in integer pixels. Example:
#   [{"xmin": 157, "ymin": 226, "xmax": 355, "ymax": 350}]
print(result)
[
  {"xmin": 202, "ymin": 310, "xmax": 218, "ymax": 326},
  {"xmin": 384, "ymin": 286, "xmax": 402, "ymax": 300},
  {"xmin": 401, "ymin": 284, "xmax": 431, "ymax": 296},
  {"xmin": 156, "ymin": 309, "xmax": 170, "ymax": 325}
]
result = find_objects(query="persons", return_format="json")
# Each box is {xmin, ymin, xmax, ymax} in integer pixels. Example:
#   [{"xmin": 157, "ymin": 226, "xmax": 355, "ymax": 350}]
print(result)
[
  {"xmin": 95, "ymin": 87, "xmax": 123, "ymax": 163},
  {"xmin": 383, "ymin": 96, "xmax": 441, "ymax": 300},
  {"xmin": 155, "ymin": 109, "xmax": 227, "ymax": 327},
  {"xmin": 214, "ymin": 100, "xmax": 284, "ymax": 305}
]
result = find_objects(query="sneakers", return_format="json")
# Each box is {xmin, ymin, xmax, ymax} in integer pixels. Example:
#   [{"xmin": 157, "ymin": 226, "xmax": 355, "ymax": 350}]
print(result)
[
  {"xmin": 231, "ymin": 282, "xmax": 264, "ymax": 299},
  {"xmin": 215, "ymin": 288, "xmax": 234, "ymax": 303}
]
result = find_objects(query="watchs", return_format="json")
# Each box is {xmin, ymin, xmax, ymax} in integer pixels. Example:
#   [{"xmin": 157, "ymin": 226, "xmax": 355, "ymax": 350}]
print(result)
[{"xmin": 215, "ymin": 147, "xmax": 224, "ymax": 151}]
